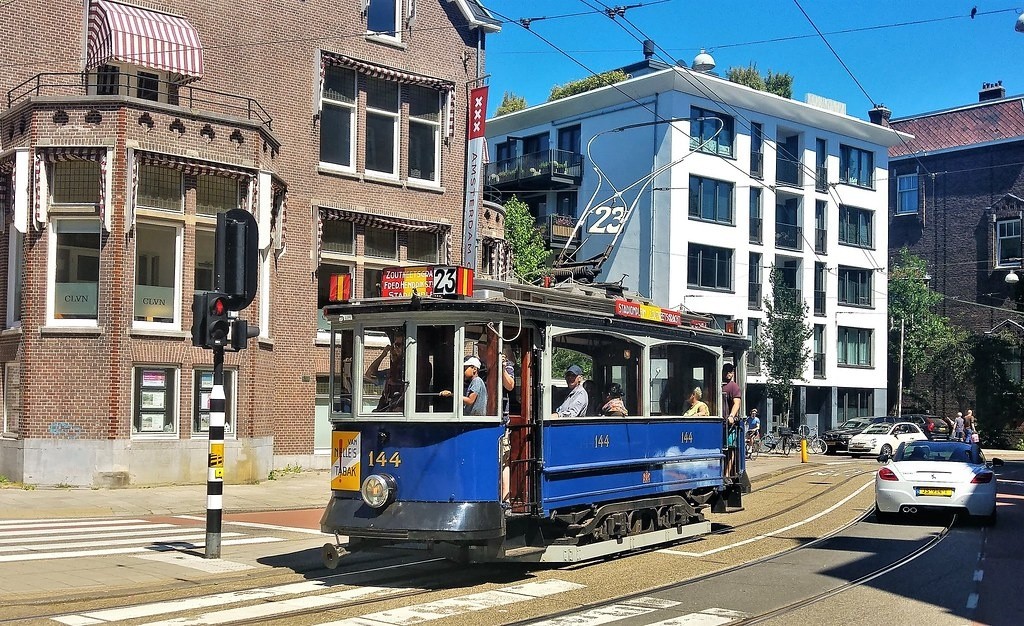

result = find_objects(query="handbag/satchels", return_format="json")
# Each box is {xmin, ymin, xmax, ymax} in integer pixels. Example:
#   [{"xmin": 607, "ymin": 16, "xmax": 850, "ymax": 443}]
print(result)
[{"xmin": 971, "ymin": 433, "xmax": 980, "ymax": 443}]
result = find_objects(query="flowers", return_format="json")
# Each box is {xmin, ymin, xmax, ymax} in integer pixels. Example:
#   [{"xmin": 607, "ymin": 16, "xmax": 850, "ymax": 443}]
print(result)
[
  {"xmin": 551, "ymin": 213, "xmax": 575, "ymax": 227},
  {"xmin": 489, "ymin": 161, "xmax": 568, "ymax": 183}
]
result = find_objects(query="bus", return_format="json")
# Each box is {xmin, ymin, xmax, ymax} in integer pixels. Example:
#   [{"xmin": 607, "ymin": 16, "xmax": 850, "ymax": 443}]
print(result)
[{"xmin": 319, "ymin": 118, "xmax": 754, "ymax": 571}]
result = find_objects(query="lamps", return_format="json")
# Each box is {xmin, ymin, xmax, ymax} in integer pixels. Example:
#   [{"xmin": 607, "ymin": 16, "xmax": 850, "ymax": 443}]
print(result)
[
  {"xmin": 691, "ymin": 49, "xmax": 716, "ymax": 73},
  {"xmin": 1004, "ymin": 269, "xmax": 1019, "ymax": 283},
  {"xmin": 1014, "ymin": 11, "xmax": 1024, "ymax": 33}
]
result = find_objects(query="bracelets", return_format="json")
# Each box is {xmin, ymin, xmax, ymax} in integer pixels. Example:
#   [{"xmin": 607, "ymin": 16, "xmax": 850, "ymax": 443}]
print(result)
[{"xmin": 730, "ymin": 414, "xmax": 734, "ymax": 418}]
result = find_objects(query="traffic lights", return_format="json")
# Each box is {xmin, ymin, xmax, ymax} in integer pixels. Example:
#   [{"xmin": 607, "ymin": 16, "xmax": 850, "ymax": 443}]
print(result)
[{"xmin": 205, "ymin": 292, "xmax": 229, "ymax": 346}]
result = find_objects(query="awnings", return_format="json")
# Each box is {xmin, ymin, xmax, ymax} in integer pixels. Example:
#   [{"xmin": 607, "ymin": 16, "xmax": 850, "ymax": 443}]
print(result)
[{"xmin": 86, "ymin": 0, "xmax": 204, "ymax": 92}]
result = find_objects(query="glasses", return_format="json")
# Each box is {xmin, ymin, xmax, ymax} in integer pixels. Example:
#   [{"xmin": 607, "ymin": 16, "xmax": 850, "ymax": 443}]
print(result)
[{"xmin": 394, "ymin": 342, "xmax": 404, "ymax": 349}]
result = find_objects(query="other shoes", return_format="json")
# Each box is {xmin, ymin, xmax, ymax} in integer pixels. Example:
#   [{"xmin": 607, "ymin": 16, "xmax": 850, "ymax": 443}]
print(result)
[{"xmin": 747, "ymin": 448, "xmax": 753, "ymax": 453}]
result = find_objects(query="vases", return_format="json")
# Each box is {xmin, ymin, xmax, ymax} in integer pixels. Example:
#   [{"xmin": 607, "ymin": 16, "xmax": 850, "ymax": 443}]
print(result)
[
  {"xmin": 541, "ymin": 168, "xmax": 549, "ymax": 173},
  {"xmin": 556, "ymin": 168, "xmax": 565, "ymax": 174},
  {"xmin": 548, "ymin": 166, "xmax": 557, "ymax": 172},
  {"xmin": 552, "ymin": 224, "xmax": 576, "ymax": 237}
]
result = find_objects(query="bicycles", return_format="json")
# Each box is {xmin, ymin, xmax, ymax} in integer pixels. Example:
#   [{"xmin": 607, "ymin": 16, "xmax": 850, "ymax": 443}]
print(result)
[{"xmin": 745, "ymin": 425, "xmax": 828, "ymax": 462}]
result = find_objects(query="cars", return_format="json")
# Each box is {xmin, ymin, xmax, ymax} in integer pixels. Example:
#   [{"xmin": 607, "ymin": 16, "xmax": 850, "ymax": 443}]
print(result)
[
  {"xmin": 819, "ymin": 413, "xmax": 953, "ymax": 456},
  {"xmin": 847, "ymin": 421, "xmax": 931, "ymax": 460}
]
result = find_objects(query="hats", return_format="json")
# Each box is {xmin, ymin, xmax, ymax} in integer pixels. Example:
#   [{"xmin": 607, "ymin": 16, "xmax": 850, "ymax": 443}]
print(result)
[
  {"xmin": 463, "ymin": 355, "xmax": 482, "ymax": 368},
  {"xmin": 475, "ymin": 333, "xmax": 489, "ymax": 346},
  {"xmin": 565, "ymin": 365, "xmax": 585, "ymax": 376}
]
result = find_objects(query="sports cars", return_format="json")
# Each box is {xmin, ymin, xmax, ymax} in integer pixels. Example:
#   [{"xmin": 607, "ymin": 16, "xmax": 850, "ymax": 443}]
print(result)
[{"xmin": 874, "ymin": 440, "xmax": 1005, "ymax": 527}]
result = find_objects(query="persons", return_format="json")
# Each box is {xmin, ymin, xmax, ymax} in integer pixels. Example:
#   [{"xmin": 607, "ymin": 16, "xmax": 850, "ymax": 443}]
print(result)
[
  {"xmin": 964, "ymin": 410, "xmax": 977, "ymax": 443},
  {"xmin": 365, "ymin": 333, "xmax": 432, "ymax": 412},
  {"xmin": 439, "ymin": 356, "xmax": 488, "ymax": 416},
  {"xmin": 552, "ymin": 365, "xmax": 589, "ymax": 418},
  {"xmin": 502, "ymin": 352, "xmax": 515, "ymax": 416},
  {"xmin": 583, "ymin": 380, "xmax": 629, "ymax": 417},
  {"xmin": 723, "ymin": 363, "xmax": 741, "ymax": 485},
  {"xmin": 658, "ymin": 371, "xmax": 710, "ymax": 416},
  {"xmin": 744, "ymin": 408, "xmax": 760, "ymax": 453},
  {"xmin": 952, "ymin": 412, "xmax": 965, "ymax": 442}
]
